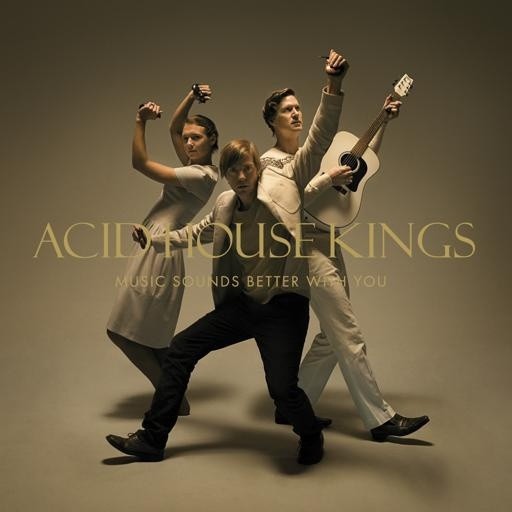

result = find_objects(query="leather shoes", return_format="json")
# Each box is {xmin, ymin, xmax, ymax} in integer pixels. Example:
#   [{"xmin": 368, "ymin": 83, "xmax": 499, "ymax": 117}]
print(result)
[
  {"xmin": 275, "ymin": 409, "xmax": 332, "ymax": 428},
  {"xmin": 105, "ymin": 431, "xmax": 164, "ymax": 462},
  {"xmin": 297, "ymin": 432, "xmax": 324, "ymax": 465},
  {"xmin": 370, "ymin": 413, "xmax": 430, "ymax": 442}
]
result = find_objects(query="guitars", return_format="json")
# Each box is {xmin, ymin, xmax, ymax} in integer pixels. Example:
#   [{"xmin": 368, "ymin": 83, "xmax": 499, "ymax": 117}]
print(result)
[{"xmin": 303, "ymin": 71, "xmax": 416, "ymax": 227}]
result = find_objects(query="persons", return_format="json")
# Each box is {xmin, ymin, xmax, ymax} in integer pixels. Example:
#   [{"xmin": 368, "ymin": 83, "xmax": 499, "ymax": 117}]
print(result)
[
  {"xmin": 107, "ymin": 84, "xmax": 219, "ymax": 415},
  {"xmin": 106, "ymin": 48, "xmax": 349, "ymax": 465},
  {"xmin": 259, "ymin": 88, "xmax": 430, "ymax": 442}
]
